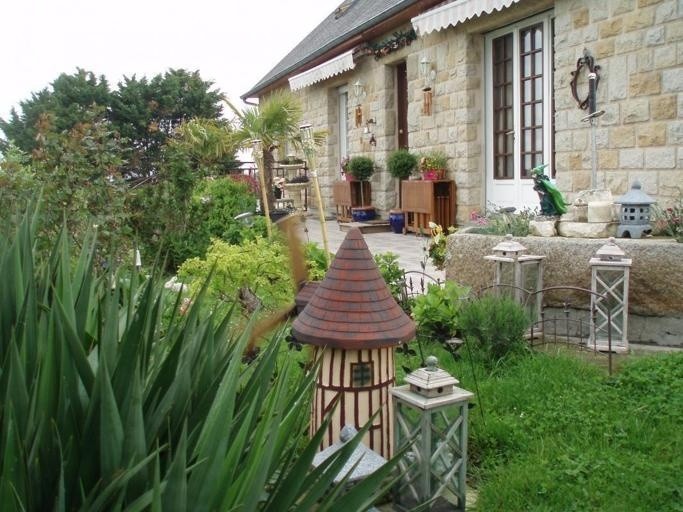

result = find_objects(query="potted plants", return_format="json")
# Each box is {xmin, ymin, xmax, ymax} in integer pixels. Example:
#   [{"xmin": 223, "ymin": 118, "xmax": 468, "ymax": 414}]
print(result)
[
  {"xmin": 339, "ymin": 155, "xmax": 376, "ymax": 222},
  {"xmin": 387, "ymin": 146, "xmax": 451, "ymax": 234},
  {"xmin": 282, "ymin": 174, "xmax": 312, "ymax": 211},
  {"xmin": 280, "ymin": 158, "xmax": 304, "ymax": 181}
]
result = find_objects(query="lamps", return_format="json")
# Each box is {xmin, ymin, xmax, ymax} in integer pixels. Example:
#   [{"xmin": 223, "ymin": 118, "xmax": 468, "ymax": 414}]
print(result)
[
  {"xmin": 420, "ymin": 54, "xmax": 436, "ymax": 92},
  {"xmin": 352, "ymin": 79, "xmax": 367, "ymax": 107}
]
